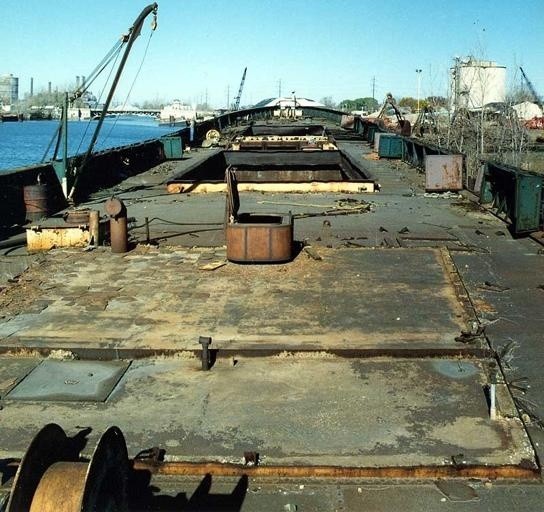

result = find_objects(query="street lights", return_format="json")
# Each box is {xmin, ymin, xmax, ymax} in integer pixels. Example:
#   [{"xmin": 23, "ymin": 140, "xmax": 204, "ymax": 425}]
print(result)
[{"xmin": 415, "ymin": 69, "xmax": 423, "ymax": 113}]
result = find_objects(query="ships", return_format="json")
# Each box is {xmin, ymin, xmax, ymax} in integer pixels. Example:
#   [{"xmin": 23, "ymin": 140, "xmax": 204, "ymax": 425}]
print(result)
[{"xmin": 0, "ymin": 105, "xmax": 543, "ymax": 509}]
